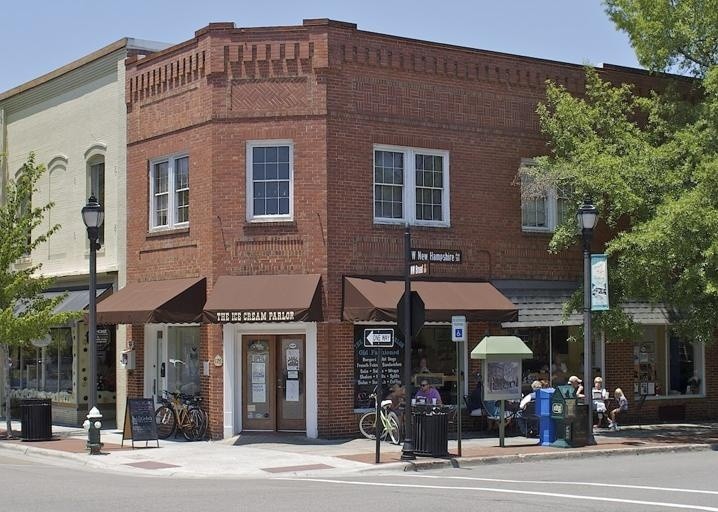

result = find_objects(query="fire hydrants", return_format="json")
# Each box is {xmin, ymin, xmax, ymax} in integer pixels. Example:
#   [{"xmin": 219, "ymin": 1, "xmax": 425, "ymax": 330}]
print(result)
[{"xmin": 83, "ymin": 405, "xmax": 103, "ymax": 455}]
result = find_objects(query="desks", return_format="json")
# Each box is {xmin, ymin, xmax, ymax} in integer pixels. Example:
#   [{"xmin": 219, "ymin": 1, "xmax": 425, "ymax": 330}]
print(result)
[{"xmin": 592, "ymin": 398, "xmax": 615, "ymax": 428}]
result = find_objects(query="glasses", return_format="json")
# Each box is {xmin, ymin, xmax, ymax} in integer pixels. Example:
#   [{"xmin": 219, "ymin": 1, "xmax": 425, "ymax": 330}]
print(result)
[{"xmin": 420, "ymin": 383, "xmax": 429, "ymax": 387}]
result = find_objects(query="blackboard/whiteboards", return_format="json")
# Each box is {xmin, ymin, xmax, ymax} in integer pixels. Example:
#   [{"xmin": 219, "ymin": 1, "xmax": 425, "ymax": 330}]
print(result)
[{"xmin": 128, "ymin": 398, "xmax": 158, "ymax": 441}]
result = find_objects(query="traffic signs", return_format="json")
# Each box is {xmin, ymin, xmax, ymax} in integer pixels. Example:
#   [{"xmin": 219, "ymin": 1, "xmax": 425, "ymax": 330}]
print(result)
[
  {"xmin": 576, "ymin": 192, "xmax": 598, "ymax": 445},
  {"xmin": 363, "ymin": 328, "xmax": 394, "ymax": 349}
]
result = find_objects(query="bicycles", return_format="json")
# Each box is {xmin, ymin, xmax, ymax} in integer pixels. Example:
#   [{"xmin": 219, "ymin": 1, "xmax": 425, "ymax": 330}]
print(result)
[
  {"xmin": 152, "ymin": 389, "xmax": 209, "ymax": 442},
  {"xmin": 359, "ymin": 386, "xmax": 400, "ymax": 447}
]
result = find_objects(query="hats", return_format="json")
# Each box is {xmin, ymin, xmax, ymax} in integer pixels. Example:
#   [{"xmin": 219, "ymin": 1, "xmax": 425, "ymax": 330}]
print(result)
[{"xmin": 568, "ymin": 375, "xmax": 583, "ymax": 383}]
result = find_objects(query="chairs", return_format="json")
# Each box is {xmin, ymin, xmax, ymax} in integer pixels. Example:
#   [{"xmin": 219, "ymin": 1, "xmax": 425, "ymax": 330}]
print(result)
[
  {"xmin": 618, "ymin": 394, "xmax": 648, "ymax": 431},
  {"xmin": 463, "ymin": 394, "xmax": 539, "ymax": 439}
]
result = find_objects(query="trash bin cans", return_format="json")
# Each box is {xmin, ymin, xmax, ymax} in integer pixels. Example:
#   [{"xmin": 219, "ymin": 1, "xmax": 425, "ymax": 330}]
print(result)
[
  {"xmin": 20, "ymin": 397, "xmax": 52, "ymax": 442},
  {"xmin": 412, "ymin": 404, "xmax": 449, "ymax": 458}
]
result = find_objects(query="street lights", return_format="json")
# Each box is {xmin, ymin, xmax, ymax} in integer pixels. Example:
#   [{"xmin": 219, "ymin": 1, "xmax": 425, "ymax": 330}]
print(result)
[{"xmin": 80, "ymin": 192, "xmax": 105, "ymax": 453}]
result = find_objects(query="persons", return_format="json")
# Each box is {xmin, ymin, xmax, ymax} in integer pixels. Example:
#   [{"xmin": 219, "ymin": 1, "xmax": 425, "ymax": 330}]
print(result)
[
  {"xmin": 472, "ymin": 375, "xmax": 550, "ymax": 436},
  {"xmin": 608, "ymin": 388, "xmax": 628, "ymax": 431},
  {"xmin": 411, "ymin": 357, "xmax": 431, "ymax": 383},
  {"xmin": 415, "ymin": 379, "xmax": 442, "ymax": 404},
  {"xmin": 591, "ymin": 376, "xmax": 607, "ymax": 428},
  {"xmin": 386, "ymin": 384, "xmax": 404, "ymax": 419},
  {"xmin": 567, "ymin": 375, "xmax": 584, "ymax": 397}
]
